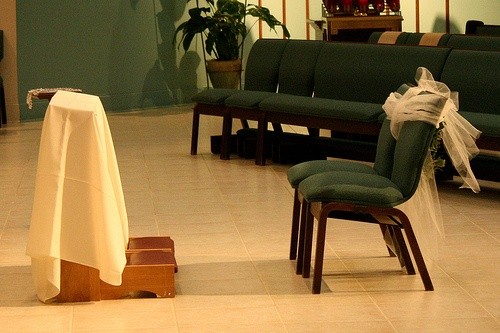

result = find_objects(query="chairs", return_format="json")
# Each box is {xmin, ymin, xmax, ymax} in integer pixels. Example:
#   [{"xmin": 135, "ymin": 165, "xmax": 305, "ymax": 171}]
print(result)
[{"xmin": 287, "ymin": 83, "xmax": 448, "ymax": 295}]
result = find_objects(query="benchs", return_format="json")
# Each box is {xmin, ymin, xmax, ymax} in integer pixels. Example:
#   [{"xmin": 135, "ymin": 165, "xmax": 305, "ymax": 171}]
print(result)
[{"xmin": 190, "ymin": 20, "xmax": 497, "ymax": 183}]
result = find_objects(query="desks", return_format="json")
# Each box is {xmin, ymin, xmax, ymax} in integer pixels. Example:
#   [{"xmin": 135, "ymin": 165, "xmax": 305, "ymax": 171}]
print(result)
[
  {"xmin": 307, "ymin": 17, "xmax": 404, "ymax": 42},
  {"xmin": 31, "ymin": 89, "xmax": 82, "ymax": 102}
]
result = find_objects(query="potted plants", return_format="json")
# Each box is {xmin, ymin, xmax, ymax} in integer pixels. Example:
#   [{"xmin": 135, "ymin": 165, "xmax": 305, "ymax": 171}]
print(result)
[{"xmin": 172, "ymin": 0, "xmax": 291, "ymax": 90}]
